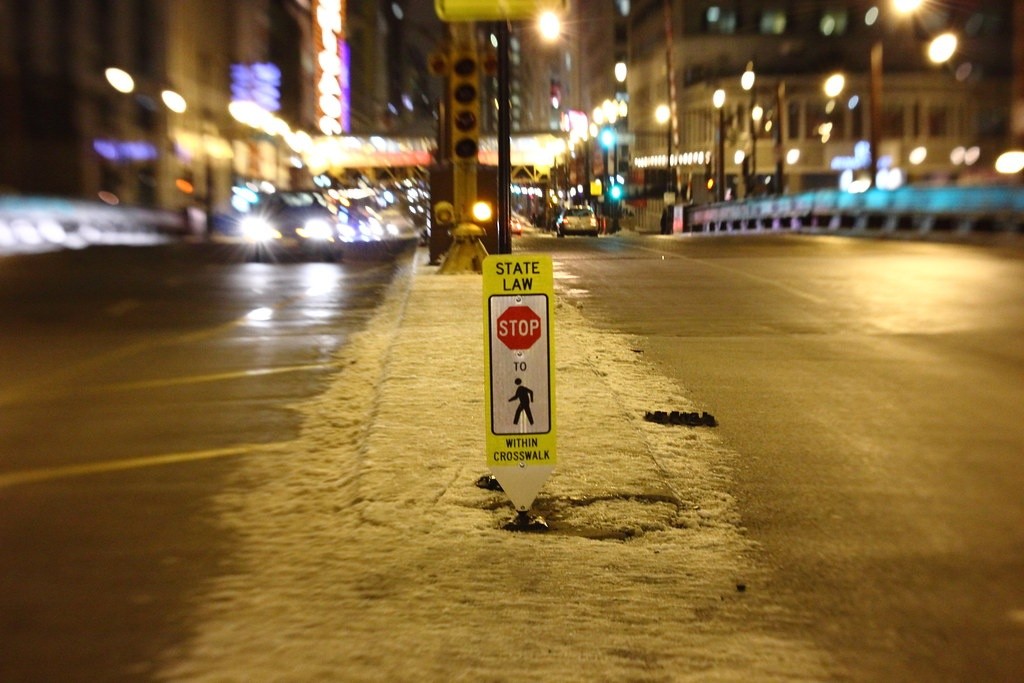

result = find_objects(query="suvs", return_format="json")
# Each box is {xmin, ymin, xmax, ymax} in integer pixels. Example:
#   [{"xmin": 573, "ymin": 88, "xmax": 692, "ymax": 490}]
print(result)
[{"xmin": 556, "ymin": 205, "xmax": 599, "ymax": 238}]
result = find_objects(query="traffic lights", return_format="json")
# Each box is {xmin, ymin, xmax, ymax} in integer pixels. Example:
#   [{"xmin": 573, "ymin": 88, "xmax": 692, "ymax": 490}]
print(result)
[{"xmin": 447, "ymin": 49, "xmax": 481, "ymax": 165}]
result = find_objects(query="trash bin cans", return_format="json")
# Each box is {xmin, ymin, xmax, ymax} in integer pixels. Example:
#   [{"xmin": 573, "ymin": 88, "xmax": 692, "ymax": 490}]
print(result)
[{"xmin": 599, "ymin": 215, "xmax": 613, "ymax": 234}]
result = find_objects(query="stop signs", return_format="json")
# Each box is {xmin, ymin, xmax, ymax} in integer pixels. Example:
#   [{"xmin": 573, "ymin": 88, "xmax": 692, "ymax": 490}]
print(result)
[{"xmin": 482, "ymin": 253, "xmax": 558, "ymax": 529}]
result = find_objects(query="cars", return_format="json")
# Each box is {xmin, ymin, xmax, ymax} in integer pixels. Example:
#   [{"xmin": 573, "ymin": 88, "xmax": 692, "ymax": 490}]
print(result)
[
  {"xmin": 246, "ymin": 190, "xmax": 337, "ymax": 260},
  {"xmin": 511, "ymin": 218, "xmax": 521, "ymax": 235}
]
